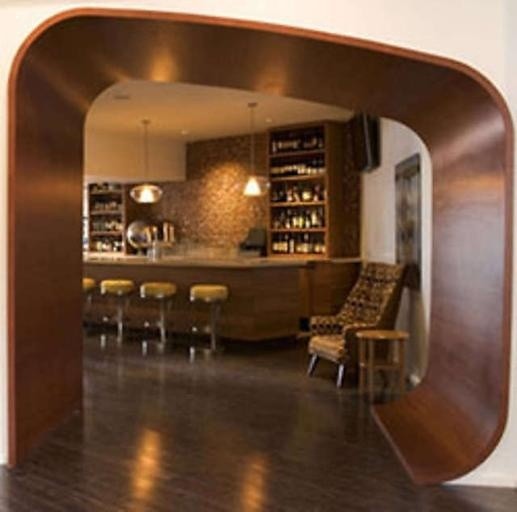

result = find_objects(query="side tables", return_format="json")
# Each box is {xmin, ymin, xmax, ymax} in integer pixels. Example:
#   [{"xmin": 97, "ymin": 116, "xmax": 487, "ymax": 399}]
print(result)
[{"xmin": 356, "ymin": 330, "xmax": 410, "ymax": 403}]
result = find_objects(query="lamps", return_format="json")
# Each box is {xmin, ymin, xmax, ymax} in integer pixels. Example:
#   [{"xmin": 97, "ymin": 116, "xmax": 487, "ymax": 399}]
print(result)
[
  {"xmin": 129, "ymin": 119, "xmax": 164, "ymax": 204},
  {"xmin": 235, "ymin": 102, "xmax": 272, "ymax": 196}
]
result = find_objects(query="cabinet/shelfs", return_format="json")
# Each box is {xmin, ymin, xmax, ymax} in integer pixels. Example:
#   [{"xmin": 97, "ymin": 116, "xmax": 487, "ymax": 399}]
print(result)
[
  {"xmin": 264, "ymin": 119, "xmax": 361, "ymax": 259},
  {"xmin": 88, "ymin": 183, "xmax": 151, "ymax": 255}
]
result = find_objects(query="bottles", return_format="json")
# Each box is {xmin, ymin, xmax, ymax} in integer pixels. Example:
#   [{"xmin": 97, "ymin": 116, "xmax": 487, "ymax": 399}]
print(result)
[{"xmin": 271, "ymin": 132, "xmax": 326, "ymax": 254}]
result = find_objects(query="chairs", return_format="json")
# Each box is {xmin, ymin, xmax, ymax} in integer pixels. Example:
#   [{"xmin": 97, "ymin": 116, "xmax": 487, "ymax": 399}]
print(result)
[{"xmin": 307, "ymin": 262, "xmax": 408, "ymax": 388}]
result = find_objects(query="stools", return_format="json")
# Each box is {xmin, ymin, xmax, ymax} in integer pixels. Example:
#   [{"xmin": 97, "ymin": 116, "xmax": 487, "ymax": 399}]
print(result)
[
  {"xmin": 189, "ymin": 284, "xmax": 228, "ymax": 363},
  {"xmin": 139, "ymin": 282, "xmax": 177, "ymax": 356},
  {"xmin": 83, "ymin": 277, "xmax": 96, "ymax": 339},
  {"xmin": 100, "ymin": 278, "xmax": 135, "ymax": 349}
]
